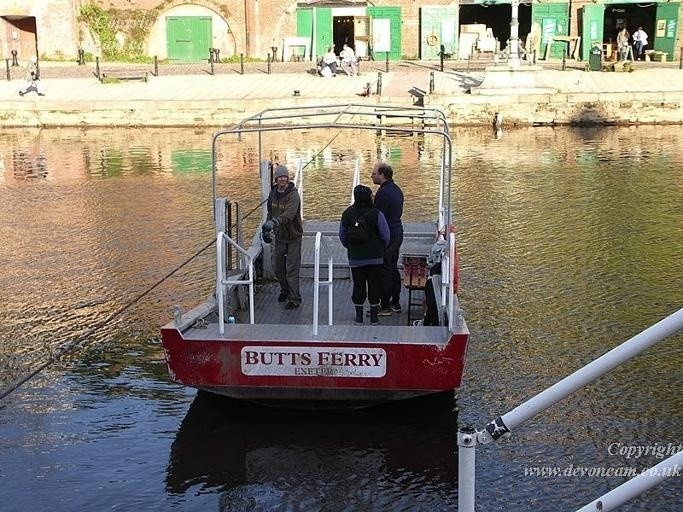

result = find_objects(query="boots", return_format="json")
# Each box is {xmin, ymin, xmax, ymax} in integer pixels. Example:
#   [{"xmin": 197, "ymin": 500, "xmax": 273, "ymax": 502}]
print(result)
[
  {"xmin": 353, "ymin": 304, "xmax": 363, "ymax": 326},
  {"xmin": 370, "ymin": 304, "xmax": 381, "ymax": 326}
]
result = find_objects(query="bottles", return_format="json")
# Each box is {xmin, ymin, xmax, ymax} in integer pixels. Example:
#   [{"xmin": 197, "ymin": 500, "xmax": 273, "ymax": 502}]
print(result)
[{"xmin": 173, "ymin": 304, "xmax": 181, "ymax": 326}]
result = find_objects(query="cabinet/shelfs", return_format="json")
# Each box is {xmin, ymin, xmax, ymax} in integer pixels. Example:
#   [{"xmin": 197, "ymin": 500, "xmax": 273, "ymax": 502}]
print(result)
[{"xmin": 601, "ymin": 43, "xmax": 612, "ymax": 60}]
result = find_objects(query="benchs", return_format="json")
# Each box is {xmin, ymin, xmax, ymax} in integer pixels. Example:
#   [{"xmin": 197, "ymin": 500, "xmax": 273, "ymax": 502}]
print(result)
[
  {"xmin": 100, "ymin": 66, "xmax": 151, "ymax": 82},
  {"xmin": 314, "ymin": 56, "xmax": 364, "ymax": 78},
  {"xmin": 401, "ymin": 253, "xmax": 433, "ymax": 326},
  {"xmin": 642, "ymin": 51, "xmax": 668, "ymax": 62}
]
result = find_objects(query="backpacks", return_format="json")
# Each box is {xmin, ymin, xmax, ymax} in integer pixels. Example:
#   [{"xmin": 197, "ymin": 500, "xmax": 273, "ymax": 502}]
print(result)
[{"xmin": 348, "ymin": 206, "xmax": 377, "ymax": 244}]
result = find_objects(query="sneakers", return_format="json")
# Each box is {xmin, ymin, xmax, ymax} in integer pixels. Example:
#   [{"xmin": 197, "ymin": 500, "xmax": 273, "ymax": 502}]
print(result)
[
  {"xmin": 278, "ymin": 294, "xmax": 287, "ymax": 302},
  {"xmin": 365, "ymin": 300, "xmax": 401, "ymax": 316},
  {"xmin": 285, "ymin": 302, "xmax": 299, "ymax": 309}
]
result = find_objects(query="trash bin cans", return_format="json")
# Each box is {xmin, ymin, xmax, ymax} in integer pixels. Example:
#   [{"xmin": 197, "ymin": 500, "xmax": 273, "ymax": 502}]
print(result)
[{"xmin": 589, "ymin": 46, "xmax": 602, "ymax": 71}]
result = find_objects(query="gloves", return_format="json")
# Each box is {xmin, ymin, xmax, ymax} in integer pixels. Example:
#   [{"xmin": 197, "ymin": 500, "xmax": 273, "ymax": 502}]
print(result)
[{"xmin": 262, "ymin": 218, "xmax": 280, "ymax": 243}]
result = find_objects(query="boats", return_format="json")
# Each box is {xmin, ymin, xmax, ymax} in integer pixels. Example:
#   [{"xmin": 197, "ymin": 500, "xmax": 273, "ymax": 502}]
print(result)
[
  {"xmin": 163, "ymin": 389, "xmax": 459, "ymax": 493},
  {"xmin": 157, "ymin": 103, "xmax": 471, "ymax": 412}
]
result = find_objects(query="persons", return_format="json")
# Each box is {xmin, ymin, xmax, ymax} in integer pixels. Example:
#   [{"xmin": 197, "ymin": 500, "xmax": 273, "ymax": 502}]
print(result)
[
  {"xmin": 323, "ymin": 47, "xmax": 339, "ymax": 77},
  {"xmin": 262, "ymin": 165, "xmax": 302, "ymax": 309},
  {"xmin": 632, "ymin": 27, "xmax": 648, "ymax": 60},
  {"xmin": 617, "ymin": 26, "xmax": 629, "ymax": 60},
  {"xmin": 19, "ymin": 55, "xmax": 45, "ymax": 96},
  {"xmin": 340, "ymin": 44, "xmax": 356, "ymax": 77},
  {"xmin": 339, "ymin": 185, "xmax": 391, "ymax": 325},
  {"xmin": 370, "ymin": 163, "xmax": 404, "ymax": 317}
]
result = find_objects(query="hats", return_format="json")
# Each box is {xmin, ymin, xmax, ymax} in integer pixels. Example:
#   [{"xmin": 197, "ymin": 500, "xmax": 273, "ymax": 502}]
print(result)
[{"xmin": 274, "ymin": 165, "xmax": 289, "ymax": 183}]
